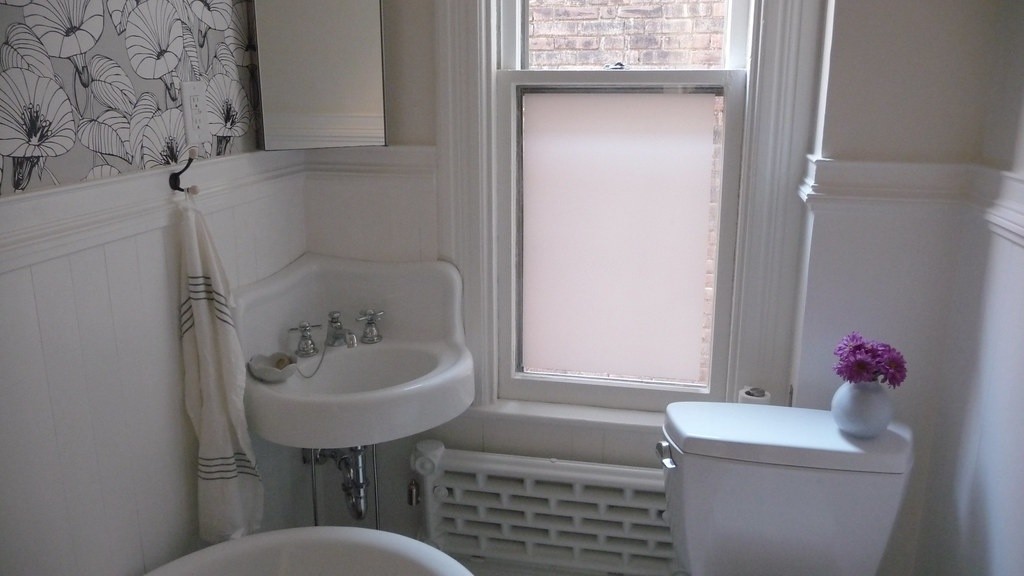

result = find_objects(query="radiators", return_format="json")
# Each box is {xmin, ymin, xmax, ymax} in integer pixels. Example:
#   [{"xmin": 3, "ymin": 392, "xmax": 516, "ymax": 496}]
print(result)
[{"xmin": 415, "ymin": 440, "xmax": 676, "ymax": 576}]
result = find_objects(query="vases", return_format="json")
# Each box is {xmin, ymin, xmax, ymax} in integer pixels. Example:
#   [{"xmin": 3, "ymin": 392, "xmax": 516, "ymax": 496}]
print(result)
[{"xmin": 831, "ymin": 378, "xmax": 894, "ymax": 438}]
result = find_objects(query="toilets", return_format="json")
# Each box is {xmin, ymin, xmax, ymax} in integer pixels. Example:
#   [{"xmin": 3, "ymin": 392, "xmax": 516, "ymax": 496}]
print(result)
[{"xmin": 655, "ymin": 402, "xmax": 918, "ymax": 576}]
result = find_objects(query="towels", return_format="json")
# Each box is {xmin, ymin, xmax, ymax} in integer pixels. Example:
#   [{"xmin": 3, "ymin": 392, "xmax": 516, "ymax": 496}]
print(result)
[{"xmin": 180, "ymin": 207, "xmax": 263, "ymax": 544}]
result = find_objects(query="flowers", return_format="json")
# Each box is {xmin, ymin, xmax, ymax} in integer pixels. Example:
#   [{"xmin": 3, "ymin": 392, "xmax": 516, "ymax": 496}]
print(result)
[{"xmin": 833, "ymin": 331, "xmax": 908, "ymax": 392}]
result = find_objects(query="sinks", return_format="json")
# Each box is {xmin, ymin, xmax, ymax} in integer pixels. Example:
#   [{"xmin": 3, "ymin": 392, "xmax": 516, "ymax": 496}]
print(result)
[
  {"xmin": 249, "ymin": 339, "xmax": 477, "ymax": 451},
  {"xmin": 146, "ymin": 523, "xmax": 475, "ymax": 576}
]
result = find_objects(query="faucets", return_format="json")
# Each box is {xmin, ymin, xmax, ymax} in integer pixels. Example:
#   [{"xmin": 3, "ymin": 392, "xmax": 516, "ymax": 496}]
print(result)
[{"xmin": 324, "ymin": 310, "xmax": 359, "ymax": 350}]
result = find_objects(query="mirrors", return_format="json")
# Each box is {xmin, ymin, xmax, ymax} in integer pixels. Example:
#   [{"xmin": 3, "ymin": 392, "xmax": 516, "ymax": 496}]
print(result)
[{"xmin": 248, "ymin": 0, "xmax": 389, "ymax": 151}]
